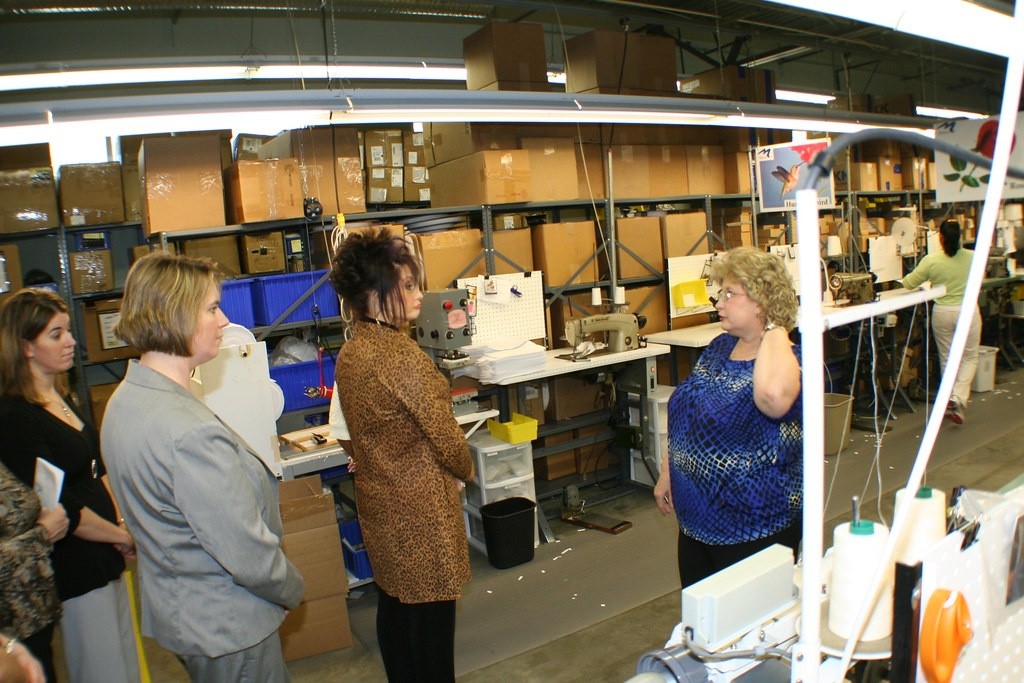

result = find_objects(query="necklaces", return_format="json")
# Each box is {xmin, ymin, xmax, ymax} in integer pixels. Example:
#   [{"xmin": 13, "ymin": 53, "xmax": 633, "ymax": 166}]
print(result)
[{"xmin": 31, "ymin": 388, "xmax": 72, "ymax": 419}]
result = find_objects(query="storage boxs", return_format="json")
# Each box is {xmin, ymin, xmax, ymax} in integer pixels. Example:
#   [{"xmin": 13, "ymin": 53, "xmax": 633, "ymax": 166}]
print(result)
[{"xmin": 0, "ymin": 22, "xmax": 976, "ymax": 661}]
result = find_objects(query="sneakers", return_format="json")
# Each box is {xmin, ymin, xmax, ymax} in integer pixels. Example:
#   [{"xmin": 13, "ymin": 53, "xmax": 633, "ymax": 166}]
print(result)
[{"xmin": 947, "ymin": 395, "xmax": 964, "ymax": 425}]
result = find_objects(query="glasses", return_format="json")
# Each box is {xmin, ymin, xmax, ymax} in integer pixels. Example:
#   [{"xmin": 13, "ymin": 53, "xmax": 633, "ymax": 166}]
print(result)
[{"xmin": 713, "ymin": 289, "xmax": 750, "ymax": 303}]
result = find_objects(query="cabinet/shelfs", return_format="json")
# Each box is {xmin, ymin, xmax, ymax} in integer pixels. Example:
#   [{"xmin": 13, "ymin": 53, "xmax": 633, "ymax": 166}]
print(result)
[
  {"xmin": 461, "ymin": 429, "xmax": 541, "ymax": 556},
  {"xmin": 624, "ymin": 382, "xmax": 677, "ymax": 487}
]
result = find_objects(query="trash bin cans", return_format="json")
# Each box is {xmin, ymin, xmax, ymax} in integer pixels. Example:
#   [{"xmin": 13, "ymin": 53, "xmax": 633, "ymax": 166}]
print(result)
[
  {"xmin": 823, "ymin": 392, "xmax": 855, "ymax": 456},
  {"xmin": 478, "ymin": 496, "xmax": 537, "ymax": 569},
  {"xmin": 970, "ymin": 345, "xmax": 1000, "ymax": 392}
]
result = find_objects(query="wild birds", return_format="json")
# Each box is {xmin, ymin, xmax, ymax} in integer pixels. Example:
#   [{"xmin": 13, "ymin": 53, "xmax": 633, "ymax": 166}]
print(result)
[{"xmin": 771, "ymin": 161, "xmax": 805, "ymax": 200}]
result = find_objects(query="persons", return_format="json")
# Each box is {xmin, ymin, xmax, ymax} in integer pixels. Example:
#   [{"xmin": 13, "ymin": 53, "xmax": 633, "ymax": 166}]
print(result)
[
  {"xmin": 326, "ymin": 233, "xmax": 476, "ymax": 683},
  {"xmin": 99, "ymin": 254, "xmax": 303, "ymax": 683},
  {"xmin": 0, "ymin": 287, "xmax": 140, "ymax": 683},
  {"xmin": 902, "ymin": 218, "xmax": 983, "ymax": 425},
  {"xmin": 654, "ymin": 247, "xmax": 803, "ymax": 591}
]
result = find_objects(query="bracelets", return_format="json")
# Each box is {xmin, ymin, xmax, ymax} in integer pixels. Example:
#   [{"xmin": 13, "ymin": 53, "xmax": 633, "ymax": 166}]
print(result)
[
  {"xmin": 116, "ymin": 518, "xmax": 128, "ymax": 524},
  {"xmin": 762, "ymin": 322, "xmax": 790, "ymax": 336},
  {"xmin": 3, "ymin": 637, "xmax": 18, "ymax": 655}
]
